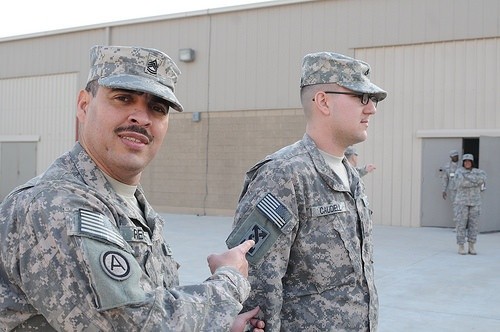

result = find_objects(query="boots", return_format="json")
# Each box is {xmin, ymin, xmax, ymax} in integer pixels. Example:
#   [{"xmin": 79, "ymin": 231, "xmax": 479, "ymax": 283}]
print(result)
[
  {"xmin": 468, "ymin": 242, "xmax": 477, "ymax": 255},
  {"xmin": 458, "ymin": 244, "xmax": 466, "ymax": 255}
]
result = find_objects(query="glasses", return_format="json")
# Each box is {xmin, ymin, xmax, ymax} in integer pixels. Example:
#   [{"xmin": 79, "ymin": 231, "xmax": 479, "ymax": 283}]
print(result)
[{"xmin": 312, "ymin": 91, "xmax": 378, "ymax": 109}]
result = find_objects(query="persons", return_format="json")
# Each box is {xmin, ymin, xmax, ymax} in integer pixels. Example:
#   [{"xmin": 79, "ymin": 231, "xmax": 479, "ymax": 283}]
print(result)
[
  {"xmin": 452, "ymin": 153, "xmax": 485, "ymax": 256},
  {"xmin": 343, "ymin": 145, "xmax": 376, "ymax": 180},
  {"xmin": 233, "ymin": 50, "xmax": 385, "ymax": 332},
  {"xmin": 442, "ymin": 150, "xmax": 463, "ymax": 201},
  {"xmin": 0, "ymin": 43, "xmax": 265, "ymax": 332}
]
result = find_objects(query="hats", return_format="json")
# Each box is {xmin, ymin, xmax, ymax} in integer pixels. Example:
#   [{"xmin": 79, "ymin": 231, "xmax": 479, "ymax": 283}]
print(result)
[
  {"xmin": 85, "ymin": 46, "xmax": 184, "ymax": 113},
  {"xmin": 462, "ymin": 153, "xmax": 473, "ymax": 161},
  {"xmin": 300, "ymin": 52, "xmax": 387, "ymax": 103},
  {"xmin": 449, "ymin": 150, "xmax": 459, "ymax": 157},
  {"xmin": 344, "ymin": 146, "xmax": 358, "ymax": 156}
]
